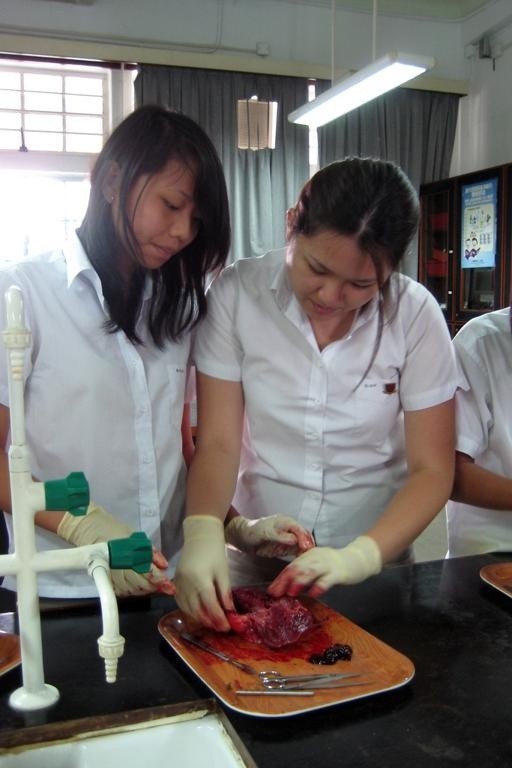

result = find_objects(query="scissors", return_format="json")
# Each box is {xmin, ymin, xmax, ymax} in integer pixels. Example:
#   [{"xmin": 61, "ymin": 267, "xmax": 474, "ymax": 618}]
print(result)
[{"xmin": 259, "ymin": 671, "xmax": 362, "ymax": 689}]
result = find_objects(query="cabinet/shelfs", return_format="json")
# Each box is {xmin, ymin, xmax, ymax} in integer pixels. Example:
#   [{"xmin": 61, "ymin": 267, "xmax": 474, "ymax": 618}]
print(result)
[{"xmin": 417, "ymin": 160, "xmax": 512, "ymax": 340}]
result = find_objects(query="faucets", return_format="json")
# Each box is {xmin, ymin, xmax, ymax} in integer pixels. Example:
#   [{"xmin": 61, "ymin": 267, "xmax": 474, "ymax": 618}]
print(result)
[{"xmin": 84, "ymin": 554, "xmax": 125, "ymax": 683}]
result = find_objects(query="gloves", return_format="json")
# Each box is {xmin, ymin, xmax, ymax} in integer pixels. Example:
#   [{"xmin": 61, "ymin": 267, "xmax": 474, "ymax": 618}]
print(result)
[
  {"xmin": 266, "ymin": 534, "xmax": 383, "ymax": 600},
  {"xmin": 174, "ymin": 513, "xmax": 239, "ymax": 633},
  {"xmin": 223, "ymin": 515, "xmax": 314, "ymax": 558},
  {"xmin": 55, "ymin": 498, "xmax": 178, "ymax": 598}
]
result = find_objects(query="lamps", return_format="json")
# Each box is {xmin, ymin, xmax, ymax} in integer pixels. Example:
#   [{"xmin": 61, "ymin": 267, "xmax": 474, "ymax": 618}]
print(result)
[{"xmin": 287, "ymin": 1, "xmax": 435, "ymax": 128}]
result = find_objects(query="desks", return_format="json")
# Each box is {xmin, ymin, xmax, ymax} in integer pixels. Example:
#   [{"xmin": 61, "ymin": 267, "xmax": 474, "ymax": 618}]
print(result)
[{"xmin": 2, "ymin": 547, "xmax": 510, "ymax": 768}]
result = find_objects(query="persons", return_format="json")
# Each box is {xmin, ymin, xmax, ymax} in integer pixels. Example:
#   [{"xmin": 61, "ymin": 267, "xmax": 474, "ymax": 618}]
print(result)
[
  {"xmin": 175, "ymin": 153, "xmax": 472, "ymax": 634},
  {"xmin": 446, "ymin": 302, "xmax": 512, "ymax": 559},
  {"xmin": 0, "ymin": 100, "xmax": 315, "ymax": 620}
]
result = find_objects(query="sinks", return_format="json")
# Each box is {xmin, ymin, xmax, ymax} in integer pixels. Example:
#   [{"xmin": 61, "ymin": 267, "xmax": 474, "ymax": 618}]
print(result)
[{"xmin": 0, "ymin": 698, "xmax": 258, "ymax": 768}]
